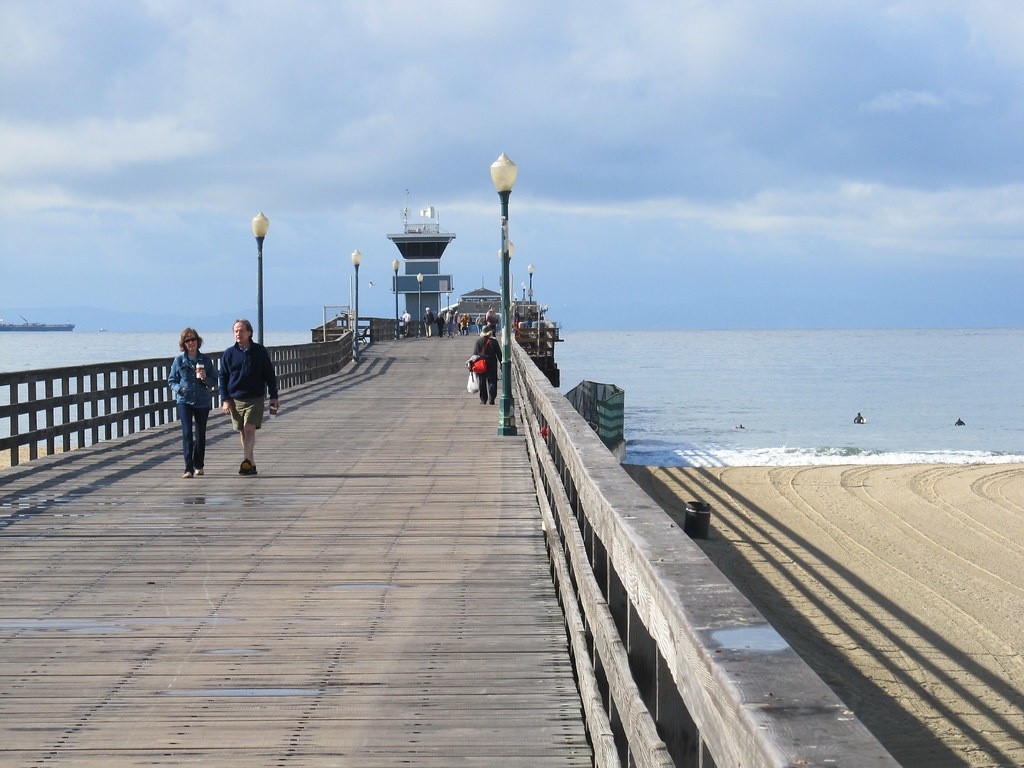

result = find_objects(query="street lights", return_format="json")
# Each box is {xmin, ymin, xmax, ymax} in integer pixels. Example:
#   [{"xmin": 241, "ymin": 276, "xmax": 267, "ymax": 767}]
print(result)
[
  {"xmin": 491, "ymin": 153, "xmax": 517, "ymax": 436},
  {"xmin": 514, "ymin": 292, "xmax": 518, "ymax": 304},
  {"xmin": 528, "ymin": 264, "xmax": 535, "ymax": 306},
  {"xmin": 252, "ymin": 211, "xmax": 269, "ymax": 344},
  {"xmin": 352, "ymin": 249, "xmax": 361, "ymax": 362},
  {"xmin": 417, "ymin": 273, "xmax": 423, "ymax": 337},
  {"xmin": 521, "ymin": 281, "xmax": 527, "ymax": 316},
  {"xmin": 446, "ymin": 293, "xmax": 450, "ymax": 309},
  {"xmin": 392, "ymin": 259, "xmax": 400, "ymax": 340}
]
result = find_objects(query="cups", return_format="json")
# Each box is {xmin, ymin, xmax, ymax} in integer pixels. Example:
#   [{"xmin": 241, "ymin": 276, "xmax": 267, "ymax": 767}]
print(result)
[
  {"xmin": 269, "ymin": 405, "xmax": 277, "ymax": 420},
  {"xmin": 196, "ymin": 365, "xmax": 204, "ymax": 378}
]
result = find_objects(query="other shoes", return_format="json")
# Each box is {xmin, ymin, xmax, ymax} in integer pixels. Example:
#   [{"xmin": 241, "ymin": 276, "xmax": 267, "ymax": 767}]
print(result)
[
  {"xmin": 195, "ymin": 469, "xmax": 204, "ymax": 475},
  {"xmin": 479, "ymin": 401, "xmax": 486, "ymax": 405},
  {"xmin": 490, "ymin": 402, "xmax": 495, "ymax": 405},
  {"xmin": 238, "ymin": 459, "xmax": 258, "ymax": 475},
  {"xmin": 182, "ymin": 473, "xmax": 193, "ymax": 478}
]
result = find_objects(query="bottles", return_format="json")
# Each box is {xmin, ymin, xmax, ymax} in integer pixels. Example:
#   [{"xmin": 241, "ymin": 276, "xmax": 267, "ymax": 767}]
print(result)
[{"xmin": 467, "ymin": 361, "xmax": 471, "ymax": 369}]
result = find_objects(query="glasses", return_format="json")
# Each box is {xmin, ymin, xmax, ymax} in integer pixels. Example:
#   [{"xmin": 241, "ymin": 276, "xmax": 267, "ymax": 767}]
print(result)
[{"xmin": 183, "ymin": 338, "xmax": 196, "ymax": 343}]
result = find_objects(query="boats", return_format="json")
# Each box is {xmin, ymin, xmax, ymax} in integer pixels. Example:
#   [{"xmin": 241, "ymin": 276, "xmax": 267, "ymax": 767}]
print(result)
[{"xmin": 0, "ymin": 314, "xmax": 76, "ymax": 331}]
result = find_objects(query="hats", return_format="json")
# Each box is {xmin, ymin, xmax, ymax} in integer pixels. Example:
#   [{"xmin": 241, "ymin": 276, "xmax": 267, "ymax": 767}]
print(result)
[
  {"xmin": 425, "ymin": 307, "xmax": 429, "ymax": 311},
  {"xmin": 480, "ymin": 325, "xmax": 493, "ymax": 337}
]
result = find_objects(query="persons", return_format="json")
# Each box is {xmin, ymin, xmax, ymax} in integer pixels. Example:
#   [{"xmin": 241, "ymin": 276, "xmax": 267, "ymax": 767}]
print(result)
[
  {"xmin": 168, "ymin": 328, "xmax": 217, "ymax": 478},
  {"xmin": 402, "ymin": 310, "xmax": 412, "ymax": 337},
  {"xmin": 736, "ymin": 425, "xmax": 745, "ymax": 429},
  {"xmin": 955, "ymin": 417, "xmax": 965, "ymax": 426},
  {"xmin": 219, "ymin": 319, "xmax": 280, "ymax": 475},
  {"xmin": 469, "ymin": 325, "xmax": 502, "ymax": 405},
  {"xmin": 854, "ymin": 413, "xmax": 864, "ymax": 424},
  {"xmin": 434, "ymin": 313, "xmax": 445, "ymax": 337},
  {"xmin": 422, "ymin": 307, "xmax": 434, "ymax": 338},
  {"xmin": 445, "ymin": 306, "xmax": 532, "ymax": 338},
  {"xmin": 486, "ymin": 304, "xmax": 498, "ymax": 339}
]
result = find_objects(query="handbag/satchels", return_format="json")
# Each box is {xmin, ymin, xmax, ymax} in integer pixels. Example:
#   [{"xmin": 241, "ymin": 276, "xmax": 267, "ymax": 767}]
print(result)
[
  {"xmin": 471, "ymin": 355, "xmax": 487, "ymax": 374},
  {"xmin": 466, "ymin": 371, "xmax": 479, "ymax": 394}
]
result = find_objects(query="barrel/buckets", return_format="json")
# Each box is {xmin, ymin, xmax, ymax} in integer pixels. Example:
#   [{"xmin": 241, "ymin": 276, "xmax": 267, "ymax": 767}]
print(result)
[{"xmin": 684, "ymin": 501, "xmax": 711, "ymax": 540}]
